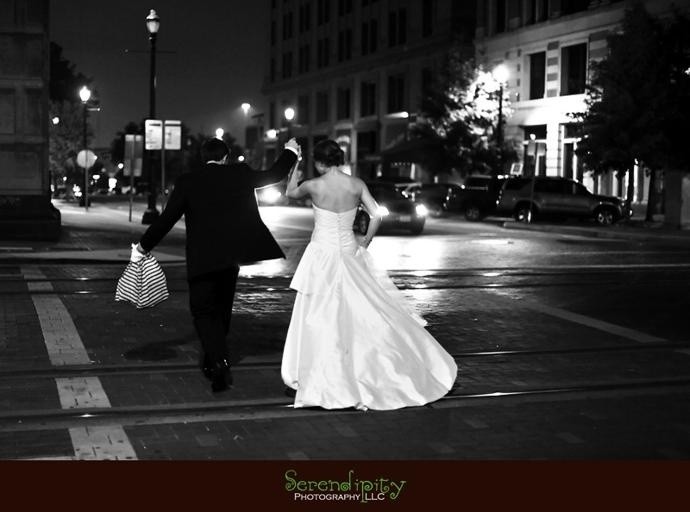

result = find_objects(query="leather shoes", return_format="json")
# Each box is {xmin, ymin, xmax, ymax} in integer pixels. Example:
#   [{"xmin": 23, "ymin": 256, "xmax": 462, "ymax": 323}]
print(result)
[{"xmin": 201, "ymin": 352, "xmax": 232, "ymax": 392}]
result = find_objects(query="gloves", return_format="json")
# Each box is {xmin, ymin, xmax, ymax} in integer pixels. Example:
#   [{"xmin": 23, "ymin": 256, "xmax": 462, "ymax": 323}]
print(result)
[{"xmin": 130, "ymin": 243, "xmax": 145, "ymax": 262}]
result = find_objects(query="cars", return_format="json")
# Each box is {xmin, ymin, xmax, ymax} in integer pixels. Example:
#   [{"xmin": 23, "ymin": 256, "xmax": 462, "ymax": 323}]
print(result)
[
  {"xmin": 353, "ymin": 180, "xmax": 430, "ymax": 236},
  {"xmin": 396, "ymin": 172, "xmax": 634, "ymax": 227}
]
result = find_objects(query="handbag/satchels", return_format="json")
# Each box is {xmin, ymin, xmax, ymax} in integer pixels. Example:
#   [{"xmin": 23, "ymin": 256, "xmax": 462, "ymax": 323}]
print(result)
[{"xmin": 115, "ymin": 254, "xmax": 170, "ymax": 309}]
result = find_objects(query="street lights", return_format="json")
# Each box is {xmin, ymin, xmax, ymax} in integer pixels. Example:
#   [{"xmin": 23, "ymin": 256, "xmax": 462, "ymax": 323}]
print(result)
[
  {"xmin": 51, "ymin": 115, "xmax": 61, "ymax": 199},
  {"xmin": 141, "ymin": 7, "xmax": 160, "ymax": 227},
  {"xmin": 77, "ymin": 84, "xmax": 93, "ymax": 212}
]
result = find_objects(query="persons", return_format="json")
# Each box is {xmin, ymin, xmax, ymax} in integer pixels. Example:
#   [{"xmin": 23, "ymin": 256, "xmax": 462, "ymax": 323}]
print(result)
[
  {"xmin": 281, "ymin": 139, "xmax": 458, "ymax": 411},
  {"xmin": 137, "ymin": 136, "xmax": 300, "ymax": 395}
]
